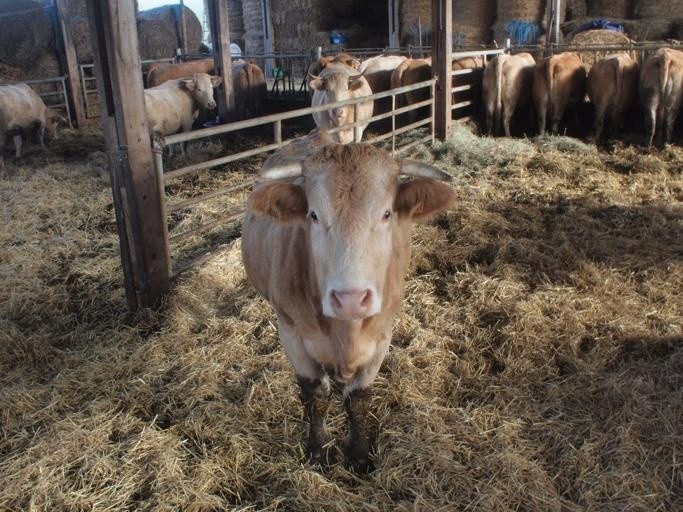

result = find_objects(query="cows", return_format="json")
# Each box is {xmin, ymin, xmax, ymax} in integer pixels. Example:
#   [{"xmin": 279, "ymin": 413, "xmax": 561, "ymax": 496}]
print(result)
[
  {"xmin": 240, "ymin": 130, "xmax": 456, "ymax": 475},
  {"xmin": 532, "ymin": 52, "xmax": 587, "ymax": 134},
  {"xmin": 481, "ymin": 51, "xmax": 536, "ymax": 136},
  {"xmin": 231, "ymin": 60, "xmax": 267, "ymax": 122},
  {"xmin": 144, "ymin": 72, "xmax": 223, "ymax": 158},
  {"xmin": 144, "ymin": 57, "xmax": 215, "ymax": 88},
  {"xmin": 307, "ymin": 50, "xmax": 486, "ymax": 141},
  {"xmin": 0, "ymin": 82, "xmax": 69, "ymax": 167},
  {"xmin": 639, "ymin": 47, "xmax": 682, "ymax": 151},
  {"xmin": 586, "ymin": 52, "xmax": 640, "ymax": 141}
]
